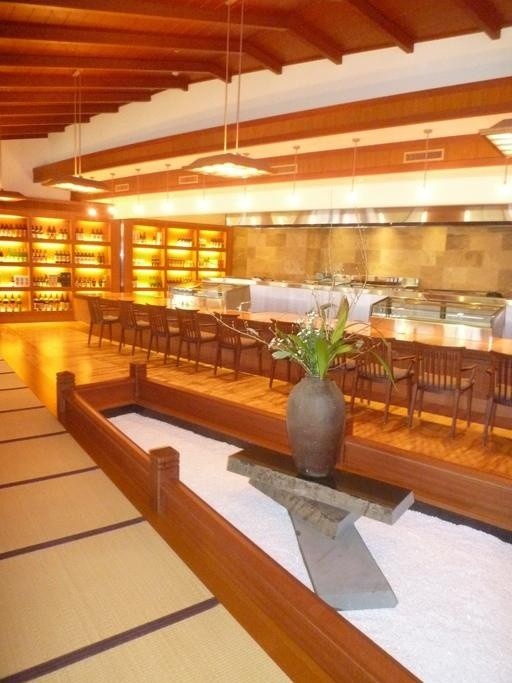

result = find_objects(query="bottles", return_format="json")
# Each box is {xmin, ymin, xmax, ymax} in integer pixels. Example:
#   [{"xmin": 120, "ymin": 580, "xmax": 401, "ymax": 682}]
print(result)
[
  {"xmin": 0, "ymin": 219, "xmax": 108, "ymax": 312},
  {"xmin": 132, "ymin": 227, "xmax": 225, "ymax": 298}
]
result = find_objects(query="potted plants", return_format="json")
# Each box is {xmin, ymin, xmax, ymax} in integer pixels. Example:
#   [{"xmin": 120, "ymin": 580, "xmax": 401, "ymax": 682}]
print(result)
[{"xmin": 214, "ymin": 269, "xmax": 398, "ymax": 481}]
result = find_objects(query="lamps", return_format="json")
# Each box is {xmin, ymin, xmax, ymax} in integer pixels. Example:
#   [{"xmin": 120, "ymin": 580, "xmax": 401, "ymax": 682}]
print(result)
[
  {"xmin": 183, "ymin": 2, "xmax": 272, "ymax": 181},
  {"xmin": 479, "ymin": 118, "xmax": 512, "ymax": 160},
  {"xmin": 44, "ymin": 67, "xmax": 112, "ymax": 195}
]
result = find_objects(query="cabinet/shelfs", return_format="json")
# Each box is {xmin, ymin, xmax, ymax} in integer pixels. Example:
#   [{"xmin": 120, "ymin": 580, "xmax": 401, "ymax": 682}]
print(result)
[{"xmin": 0, "ymin": 201, "xmax": 236, "ymax": 325}]
